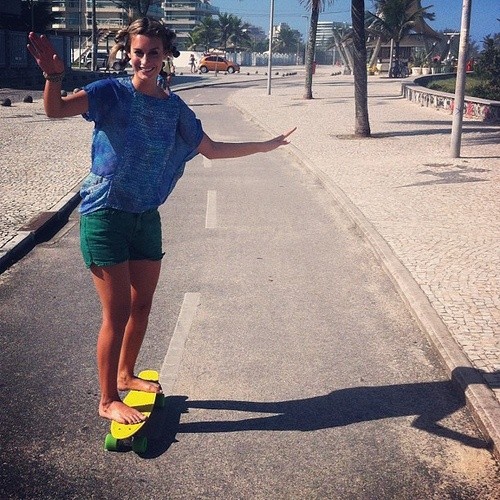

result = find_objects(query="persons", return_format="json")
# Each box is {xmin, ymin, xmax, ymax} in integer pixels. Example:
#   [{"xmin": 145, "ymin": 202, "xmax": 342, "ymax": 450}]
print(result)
[{"xmin": 28, "ymin": 19, "xmax": 297, "ymax": 424}]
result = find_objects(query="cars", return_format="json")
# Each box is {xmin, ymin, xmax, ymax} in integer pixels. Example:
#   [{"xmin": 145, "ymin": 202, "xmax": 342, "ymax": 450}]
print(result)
[{"xmin": 198, "ymin": 56, "xmax": 240, "ymax": 74}]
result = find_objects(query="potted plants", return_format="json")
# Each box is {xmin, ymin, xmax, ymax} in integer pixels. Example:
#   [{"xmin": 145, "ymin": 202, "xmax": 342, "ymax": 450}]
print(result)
[{"xmin": 409, "ymin": 50, "xmax": 452, "ymax": 75}]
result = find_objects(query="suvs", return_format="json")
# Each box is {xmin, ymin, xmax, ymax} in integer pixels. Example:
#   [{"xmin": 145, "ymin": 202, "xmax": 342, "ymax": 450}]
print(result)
[{"xmin": 83, "ymin": 52, "xmax": 122, "ymax": 71}]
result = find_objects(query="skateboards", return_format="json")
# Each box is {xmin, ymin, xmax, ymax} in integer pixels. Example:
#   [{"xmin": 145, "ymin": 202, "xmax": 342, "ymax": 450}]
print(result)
[{"xmin": 104, "ymin": 369, "xmax": 165, "ymax": 453}]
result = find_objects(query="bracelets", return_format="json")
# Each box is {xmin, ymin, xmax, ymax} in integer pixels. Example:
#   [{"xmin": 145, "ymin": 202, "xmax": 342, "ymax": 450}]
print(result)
[{"xmin": 42, "ymin": 69, "xmax": 65, "ymax": 82}]
